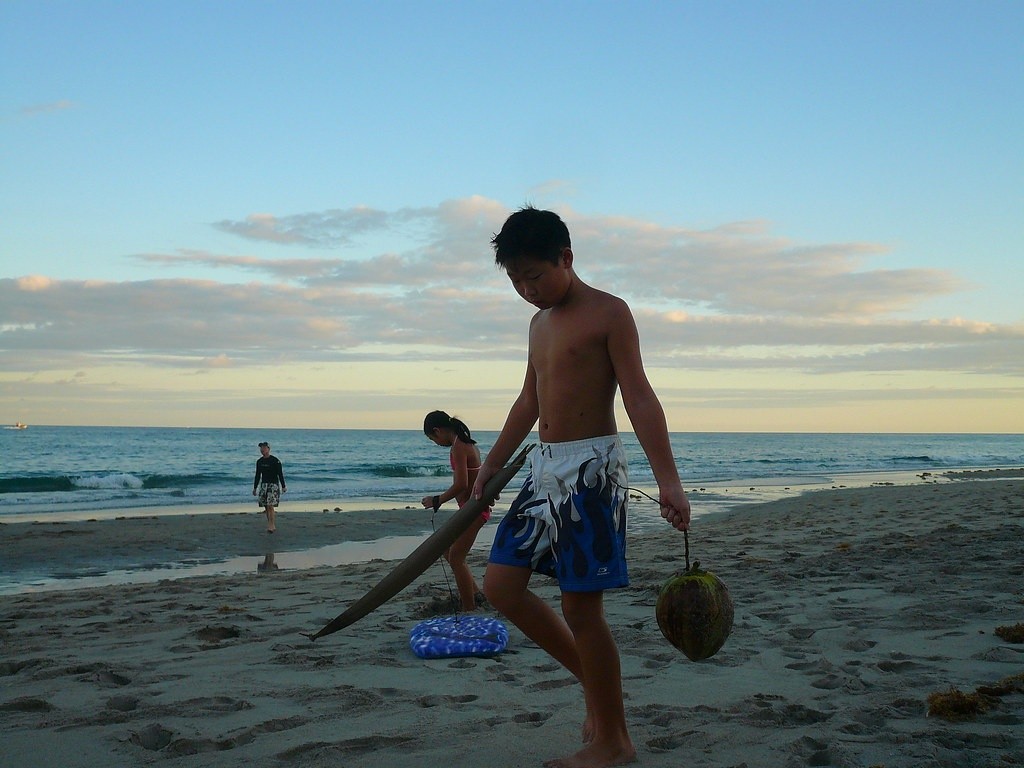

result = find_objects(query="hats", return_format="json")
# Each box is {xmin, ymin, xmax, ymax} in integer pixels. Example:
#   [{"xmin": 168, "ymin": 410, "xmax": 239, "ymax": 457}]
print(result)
[{"xmin": 258, "ymin": 441, "xmax": 271, "ymax": 450}]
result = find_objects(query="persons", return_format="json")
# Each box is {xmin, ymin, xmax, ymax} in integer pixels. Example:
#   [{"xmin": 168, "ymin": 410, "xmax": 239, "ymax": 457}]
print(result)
[
  {"xmin": 421, "ymin": 409, "xmax": 490, "ymax": 615},
  {"xmin": 465, "ymin": 203, "xmax": 691, "ymax": 767},
  {"xmin": 252, "ymin": 441, "xmax": 286, "ymax": 534}
]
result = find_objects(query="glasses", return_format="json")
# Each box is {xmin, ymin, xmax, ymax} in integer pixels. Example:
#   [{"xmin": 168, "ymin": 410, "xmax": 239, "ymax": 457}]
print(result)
[{"xmin": 258, "ymin": 442, "xmax": 268, "ymax": 447}]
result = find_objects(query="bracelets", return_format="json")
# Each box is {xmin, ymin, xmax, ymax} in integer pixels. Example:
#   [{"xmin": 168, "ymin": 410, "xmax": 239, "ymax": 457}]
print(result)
[{"xmin": 433, "ymin": 495, "xmax": 441, "ymax": 513}]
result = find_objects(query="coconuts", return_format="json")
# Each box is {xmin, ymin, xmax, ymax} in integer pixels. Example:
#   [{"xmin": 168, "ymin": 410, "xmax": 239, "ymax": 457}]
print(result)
[{"xmin": 655, "ymin": 561, "xmax": 734, "ymax": 662}]
